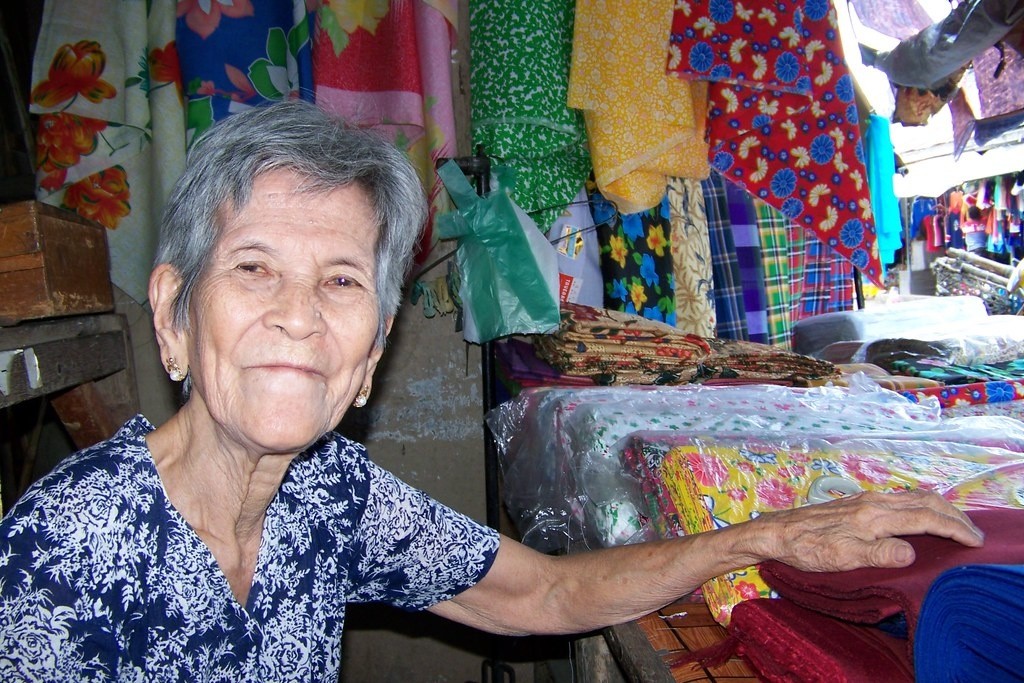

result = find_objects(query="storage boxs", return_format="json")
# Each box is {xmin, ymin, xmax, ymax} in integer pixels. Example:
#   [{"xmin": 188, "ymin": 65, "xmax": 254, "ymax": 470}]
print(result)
[{"xmin": 0, "ymin": 200, "xmax": 115, "ymax": 323}]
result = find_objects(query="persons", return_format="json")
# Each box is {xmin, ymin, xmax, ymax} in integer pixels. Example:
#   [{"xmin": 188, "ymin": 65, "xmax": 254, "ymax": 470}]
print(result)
[{"xmin": 0, "ymin": 98, "xmax": 985, "ymax": 683}]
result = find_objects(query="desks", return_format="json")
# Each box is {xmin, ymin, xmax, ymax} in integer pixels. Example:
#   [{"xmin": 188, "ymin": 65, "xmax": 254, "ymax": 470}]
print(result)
[
  {"xmin": 0, "ymin": 316, "xmax": 130, "ymax": 450},
  {"xmin": 554, "ymin": 538, "xmax": 767, "ymax": 683}
]
